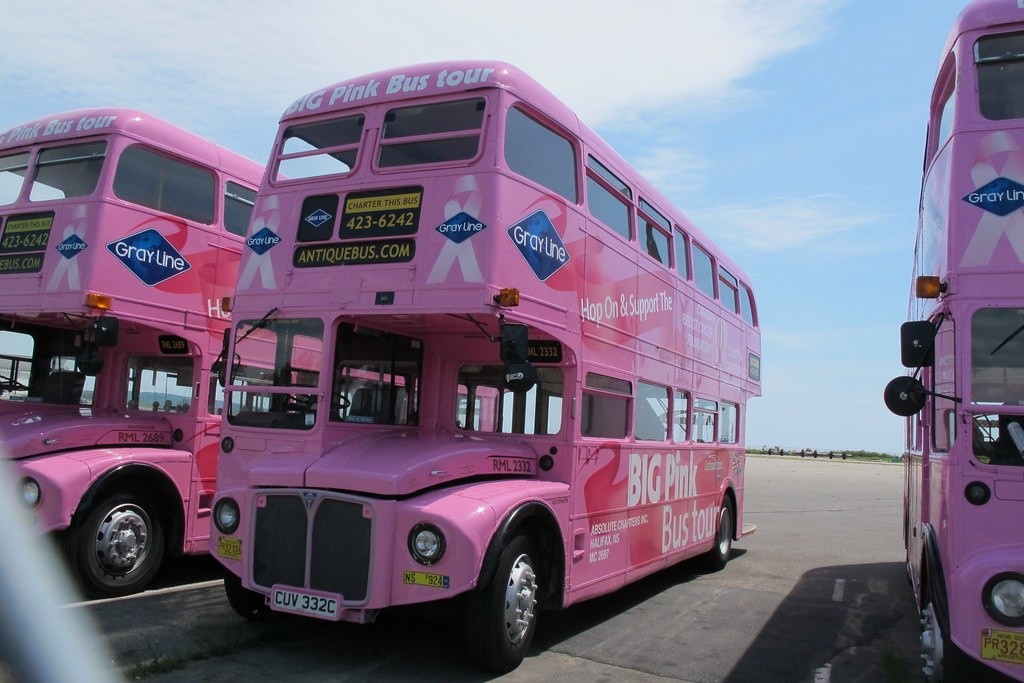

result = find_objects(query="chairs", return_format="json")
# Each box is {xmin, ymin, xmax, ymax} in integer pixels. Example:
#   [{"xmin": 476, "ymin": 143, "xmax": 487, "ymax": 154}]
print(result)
[
  {"xmin": 41, "ymin": 372, "xmax": 86, "ymax": 406},
  {"xmin": 347, "ymin": 385, "xmax": 406, "ymax": 426}
]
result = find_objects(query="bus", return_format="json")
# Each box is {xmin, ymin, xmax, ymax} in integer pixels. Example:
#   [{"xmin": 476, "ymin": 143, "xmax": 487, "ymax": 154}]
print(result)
[
  {"xmin": 203, "ymin": 55, "xmax": 766, "ymax": 672},
  {"xmin": 0, "ymin": 108, "xmax": 294, "ymax": 598},
  {"xmin": 881, "ymin": 0, "xmax": 1024, "ymax": 682}
]
